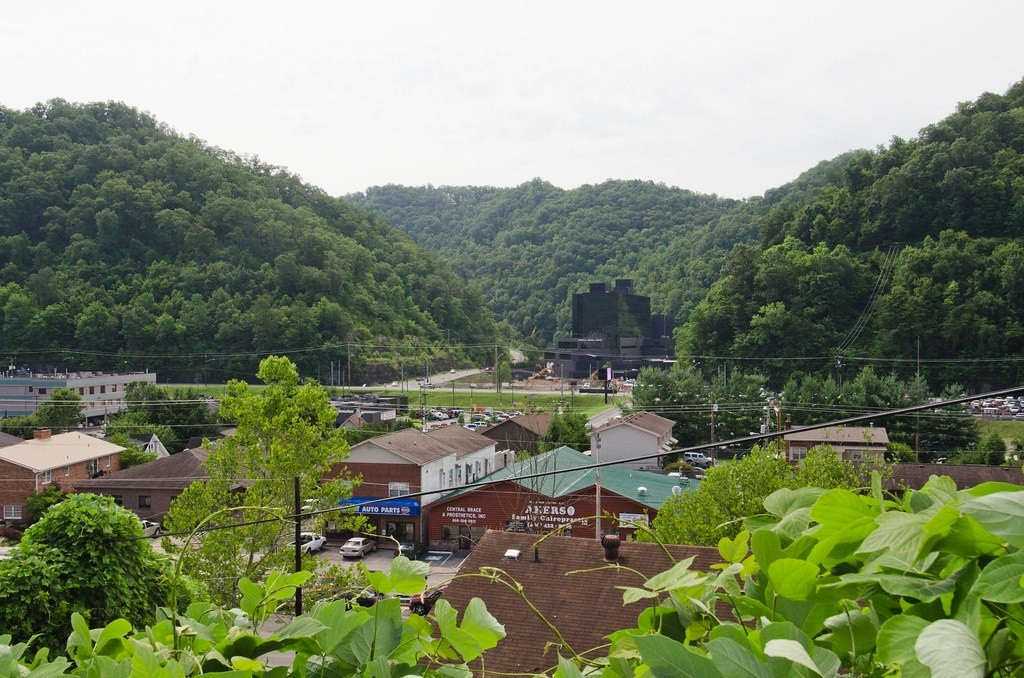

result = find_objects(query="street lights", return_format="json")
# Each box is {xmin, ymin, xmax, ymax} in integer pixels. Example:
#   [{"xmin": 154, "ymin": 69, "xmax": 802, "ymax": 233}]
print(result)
[{"xmin": 585, "ymin": 422, "xmax": 603, "ymax": 540}]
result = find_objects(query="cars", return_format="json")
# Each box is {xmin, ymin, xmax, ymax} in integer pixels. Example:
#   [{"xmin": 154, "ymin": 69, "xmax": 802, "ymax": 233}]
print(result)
[
  {"xmin": 484, "ymin": 367, "xmax": 492, "ymax": 371},
  {"xmin": 327, "ymin": 585, "xmax": 378, "ymax": 609},
  {"xmin": 139, "ymin": 519, "xmax": 160, "ymax": 538},
  {"xmin": 420, "ymin": 383, "xmax": 435, "ymax": 389},
  {"xmin": 450, "ymin": 369, "xmax": 456, "ymax": 374},
  {"xmin": 73, "ymin": 418, "xmax": 111, "ymax": 439},
  {"xmin": 339, "ymin": 537, "xmax": 378, "ymax": 559},
  {"xmin": 412, "ymin": 404, "xmax": 526, "ymax": 434},
  {"xmin": 394, "ymin": 541, "xmax": 427, "ymax": 562},
  {"xmin": 901, "ymin": 391, "xmax": 1024, "ymax": 423},
  {"xmin": 687, "ymin": 466, "xmax": 708, "ymax": 481},
  {"xmin": 391, "ymin": 380, "xmax": 400, "ymax": 388},
  {"xmin": 581, "ymin": 376, "xmax": 654, "ymax": 395}
]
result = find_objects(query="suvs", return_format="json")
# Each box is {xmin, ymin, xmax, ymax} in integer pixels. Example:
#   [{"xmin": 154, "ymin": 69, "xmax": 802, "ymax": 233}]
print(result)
[
  {"xmin": 683, "ymin": 451, "xmax": 716, "ymax": 468},
  {"xmin": 408, "ymin": 585, "xmax": 449, "ymax": 617}
]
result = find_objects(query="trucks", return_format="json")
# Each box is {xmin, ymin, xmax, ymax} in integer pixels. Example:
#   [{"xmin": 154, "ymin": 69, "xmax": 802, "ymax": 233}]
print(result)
[{"xmin": 287, "ymin": 532, "xmax": 327, "ymax": 555}]
